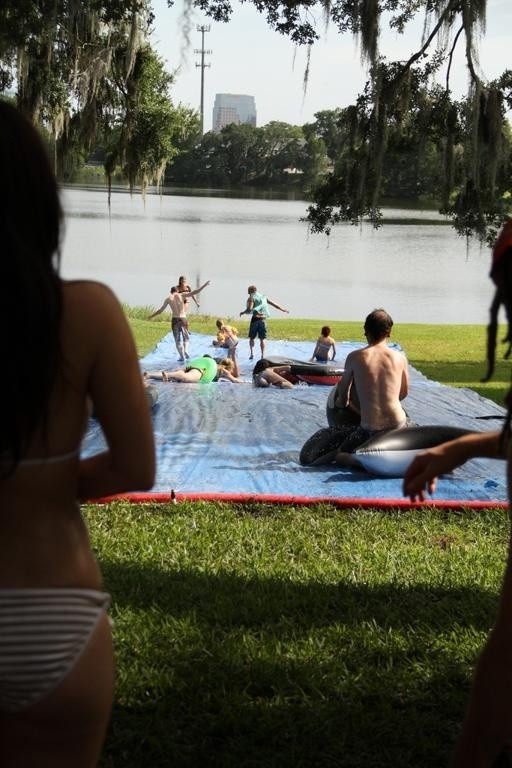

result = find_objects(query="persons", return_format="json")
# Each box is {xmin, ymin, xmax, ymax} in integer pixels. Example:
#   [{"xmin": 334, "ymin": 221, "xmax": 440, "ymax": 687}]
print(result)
[
  {"xmin": 239, "ymin": 286, "xmax": 290, "ymax": 361},
  {"xmin": 253, "ymin": 358, "xmax": 294, "ymax": 389},
  {"xmin": 2, "ymin": 97, "xmax": 160, "ymax": 765},
  {"xmin": 336, "ymin": 310, "xmax": 421, "ymax": 452},
  {"xmin": 176, "ymin": 276, "xmax": 201, "ymax": 332},
  {"xmin": 145, "ymin": 279, "xmax": 212, "ymax": 361},
  {"xmin": 307, "ymin": 326, "xmax": 336, "ymax": 362},
  {"xmin": 142, "ymin": 358, "xmax": 253, "ymax": 383},
  {"xmin": 400, "ymin": 388, "xmax": 510, "ymax": 768},
  {"xmin": 216, "ymin": 318, "xmax": 240, "ymax": 378}
]
